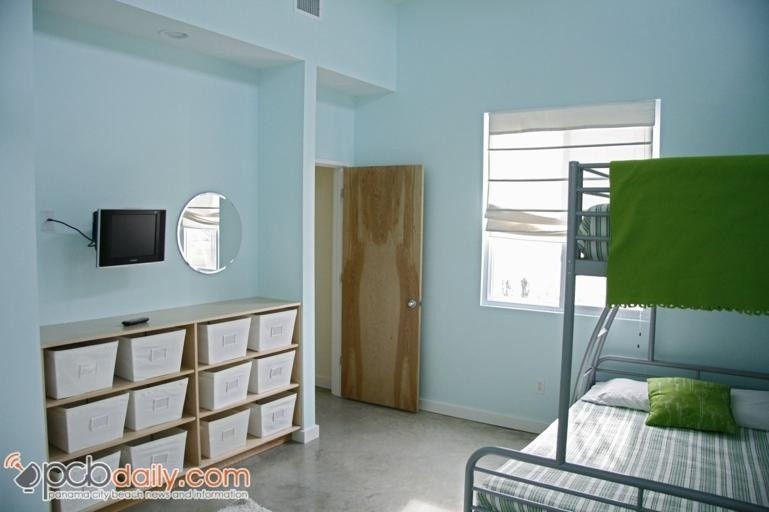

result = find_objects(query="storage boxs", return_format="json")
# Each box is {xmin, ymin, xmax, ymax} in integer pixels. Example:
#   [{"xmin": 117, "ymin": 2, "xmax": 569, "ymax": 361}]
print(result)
[
  {"xmin": 50, "ymin": 327, "xmax": 186, "ymax": 504},
  {"xmin": 200, "ymin": 311, "xmax": 296, "ymax": 459}
]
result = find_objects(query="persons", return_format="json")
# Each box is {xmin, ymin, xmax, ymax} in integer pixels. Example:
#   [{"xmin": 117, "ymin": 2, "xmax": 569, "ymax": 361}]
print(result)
[
  {"xmin": 505, "ymin": 280, "xmax": 512, "ymax": 297},
  {"xmin": 519, "ymin": 277, "xmax": 528, "ymax": 298}
]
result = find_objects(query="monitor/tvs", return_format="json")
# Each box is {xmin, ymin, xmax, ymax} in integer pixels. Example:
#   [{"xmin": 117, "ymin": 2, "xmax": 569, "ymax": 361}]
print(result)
[{"xmin": 93, "ymin": 208, "xmax": 166, "ymax": 269}]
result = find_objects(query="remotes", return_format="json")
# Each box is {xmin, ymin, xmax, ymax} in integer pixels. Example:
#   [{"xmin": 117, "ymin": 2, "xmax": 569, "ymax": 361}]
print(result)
[{"xmin": 122, "ymin": 317, "xmax": 149, "ymax": 326}]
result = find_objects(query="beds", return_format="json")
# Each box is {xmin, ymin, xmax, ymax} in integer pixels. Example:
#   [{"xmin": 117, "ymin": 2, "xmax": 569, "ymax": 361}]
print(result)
[{"xmin": 461, "ymin": 152, "xmax": 768, "ymax": 510}]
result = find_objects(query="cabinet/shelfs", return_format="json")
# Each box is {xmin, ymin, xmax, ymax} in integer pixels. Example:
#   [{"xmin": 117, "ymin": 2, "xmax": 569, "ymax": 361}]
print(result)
[{"xmin": 43, "ymin": 294, "xmax": 300, "ymax": 512}]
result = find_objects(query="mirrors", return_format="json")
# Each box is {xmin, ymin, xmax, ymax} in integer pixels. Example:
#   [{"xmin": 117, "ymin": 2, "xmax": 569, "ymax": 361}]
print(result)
[{"xmin": 175, "ymin": 191, "xmax": 243, "ymax": 273}]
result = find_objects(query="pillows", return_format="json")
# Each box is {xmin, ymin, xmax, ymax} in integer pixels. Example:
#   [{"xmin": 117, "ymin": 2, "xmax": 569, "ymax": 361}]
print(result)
[
  {"xmin": 731, "ymin": 388, "xmax": 769, "ymax": 430},
  {"xmin": 644, "ymin": 375, "xmax": 737, "ymax": 435},
  {"xmin": 579, "ymin": 375, "xmax": 648, "ymax": 412}
]
